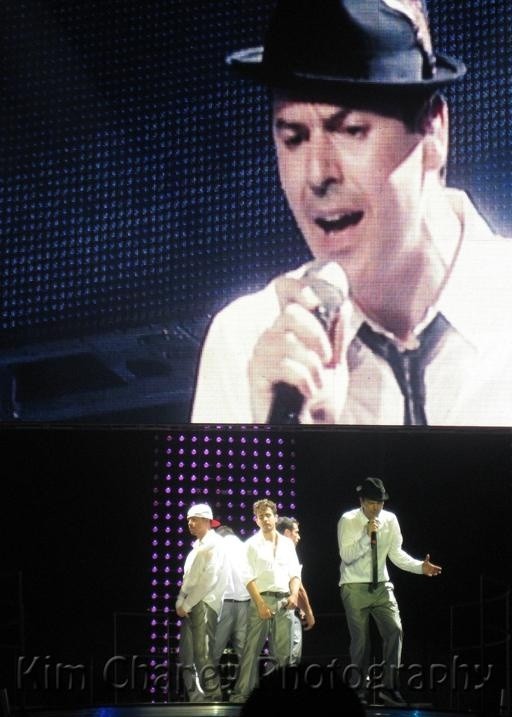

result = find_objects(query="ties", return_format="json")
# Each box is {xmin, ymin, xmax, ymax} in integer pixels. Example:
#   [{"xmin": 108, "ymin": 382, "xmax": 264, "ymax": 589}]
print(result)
[
  {"xmin": 357, "ymin": 313, "xmax": 450, "ymax": 426},
  {"xmin": 367, "ymin": 519, "xmax": 378, "ymax": 589}
]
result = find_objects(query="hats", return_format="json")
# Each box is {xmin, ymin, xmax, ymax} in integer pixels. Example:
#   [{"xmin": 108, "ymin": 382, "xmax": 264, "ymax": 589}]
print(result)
[
  {"xmin": 356, "ymin": 478, "xmax": 389, "ymax": 502},
  {"xmin": 187, "ymin": 504, "xmax": 221, "ymax": 528},
  {"xmin": 225, "ymin": 1, "xmax": 469, "ymax": 100}
]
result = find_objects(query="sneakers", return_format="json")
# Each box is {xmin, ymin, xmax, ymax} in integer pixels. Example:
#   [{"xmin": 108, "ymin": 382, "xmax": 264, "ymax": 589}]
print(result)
[
  {"xmin": 360, "ymin": 697, "xmax": 367, "ymax": 705},
  {"xmin": 379, "ymin": 688, "xmax": 405, "ymax": 707}
]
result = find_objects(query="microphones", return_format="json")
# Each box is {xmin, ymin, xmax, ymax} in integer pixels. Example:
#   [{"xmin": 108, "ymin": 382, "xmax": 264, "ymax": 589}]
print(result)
[
  {"xmin": 371, "ymin": 516, "xmax": 379, "ymax": 547},
  {"xmin": 282, "ymin": 599, "xmax": 301, "ymax": 613},
  {"xmin": 261, "ymin": 259, "xmax": 350, "ymax": 425}
]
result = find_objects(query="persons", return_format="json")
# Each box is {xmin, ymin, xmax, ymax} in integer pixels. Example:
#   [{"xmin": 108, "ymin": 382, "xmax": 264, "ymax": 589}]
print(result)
[
  {"xmin": 190, "ymin": 1, "xmax": 512, "ymax": 428},
  {"xmin": 334, "ymin": 476, "xmax": 446, "ymax": 707},
  {"xmin": 175, "ymin": 496, "xmax": 317, "ymax": 706}
]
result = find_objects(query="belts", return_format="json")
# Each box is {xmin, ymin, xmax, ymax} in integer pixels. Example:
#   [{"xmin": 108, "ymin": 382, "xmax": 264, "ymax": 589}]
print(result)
[
  {"xmin": 260, "ymin": 592, "xmax": 290, "ymax": 598},
  {"xmin": 224, "ymin": 598, "xmax": 251, "ymax": 603}
]
